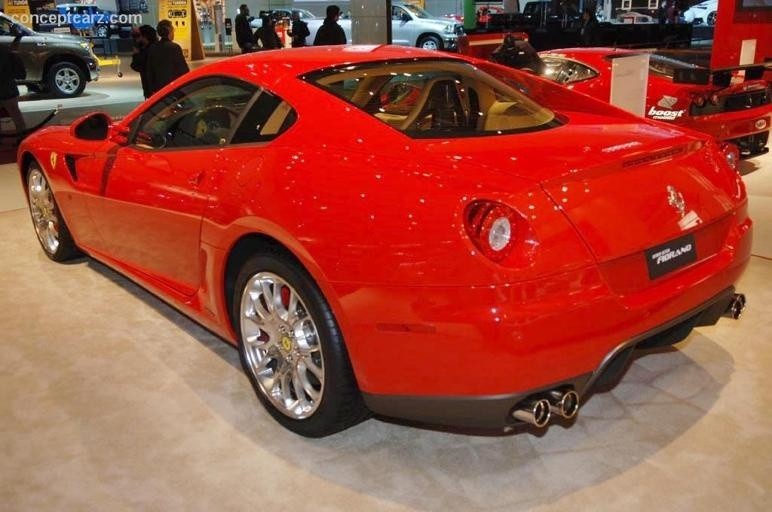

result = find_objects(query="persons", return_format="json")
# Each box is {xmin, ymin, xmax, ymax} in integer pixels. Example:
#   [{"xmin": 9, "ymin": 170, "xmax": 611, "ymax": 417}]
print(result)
[
  {"xmin": 131, "ymin": 25, "xmax": 159, "ymax": 102},
  {"xmin": 259, "ymin": 16, "xmax": 281, "ymax": 50},
  {"xmin": 287, "ymin": 14, "xmax": 310, "ymax": 48},
  {"xmin": 0, "ymin": 47, "xmax": 26, "ymax": 146},
  {"xmin": 581, "ymin": 8, "xmax": 609, "ymax": 47},
  {"xmin": 147, "ymin": 20, "xmax": 189, "ymax": 95},
  {"xmin": 235, "ymin": 5, "xmax": 259, "ymax": 54},
  {"xmin": 314, "ymin": 6, "xmax": 346, "ymax": 46}
]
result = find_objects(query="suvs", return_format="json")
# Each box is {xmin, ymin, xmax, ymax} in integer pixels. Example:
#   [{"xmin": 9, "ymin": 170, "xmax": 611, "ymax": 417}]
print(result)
[
  {"xmin": 56, "ymin": 4, "xmax": 135, "ymax": 39},
  {"xmin": 0, "ymin": 13, "xmax": 101, "ymax": 98}
]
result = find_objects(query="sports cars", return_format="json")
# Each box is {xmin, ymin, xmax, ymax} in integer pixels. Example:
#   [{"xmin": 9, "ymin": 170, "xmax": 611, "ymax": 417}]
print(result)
[
  {"xmin": 15, "ymin": 41, "xmax": 754, "ymax": 438},
  {"xmin": 476, "ymin": 46, "xmax": 770, "ymax": 162}
]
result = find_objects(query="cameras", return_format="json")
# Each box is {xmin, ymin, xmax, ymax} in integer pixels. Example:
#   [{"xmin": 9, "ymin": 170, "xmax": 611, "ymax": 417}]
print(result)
[
  {"xmin": 129, "ymin": 29, "xmax": 140, "ymax": 46},
  {"xmin": 503, "ymin": 34, "xmax": 521, "ymax": 55}
]
